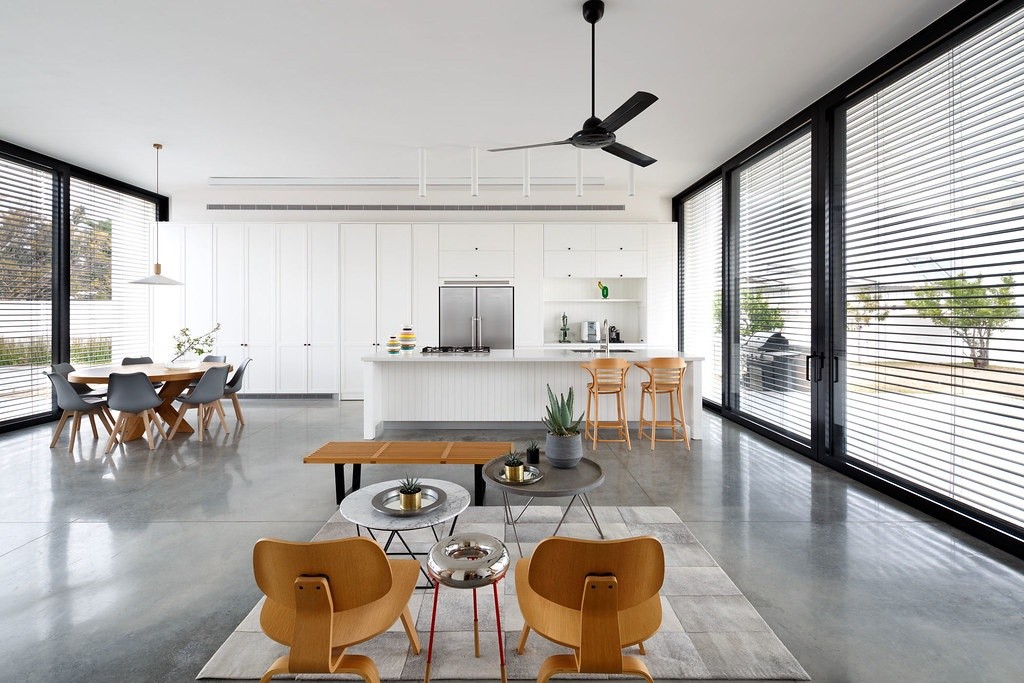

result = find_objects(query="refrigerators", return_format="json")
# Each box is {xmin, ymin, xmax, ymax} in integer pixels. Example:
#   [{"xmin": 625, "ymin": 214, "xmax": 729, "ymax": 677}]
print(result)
[{"xmin": 438, "ymin": 286, "xmax": 514, "ymax": 350}]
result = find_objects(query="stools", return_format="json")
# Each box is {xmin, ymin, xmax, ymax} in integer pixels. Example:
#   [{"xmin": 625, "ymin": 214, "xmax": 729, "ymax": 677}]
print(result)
[
  {"xmin": 580, "ymin": 358, "xmax": 632, "ymax": 452},
  {"xmin": 634, "ymin": 358, "xmax": 690, "ymax": 450}
]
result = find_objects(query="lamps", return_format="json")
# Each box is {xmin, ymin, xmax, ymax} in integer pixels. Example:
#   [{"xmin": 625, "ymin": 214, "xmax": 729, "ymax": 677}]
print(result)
[{"xmin": 130, "ymin": 144, "xmax": 183, "ymax": 285}]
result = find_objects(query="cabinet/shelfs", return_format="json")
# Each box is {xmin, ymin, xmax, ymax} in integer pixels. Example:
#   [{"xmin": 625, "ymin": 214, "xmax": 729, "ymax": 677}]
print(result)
[{"xmin": 155, "ymin": 222, "xmax": 679, "ymax": 401}]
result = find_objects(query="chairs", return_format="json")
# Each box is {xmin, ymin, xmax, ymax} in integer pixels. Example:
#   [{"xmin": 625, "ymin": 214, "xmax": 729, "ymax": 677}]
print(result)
[
  {"xmin": 252, "ymin": 536, "xmax": 420, "ymax": 683},
  {"xmin": 516, "ymin": 536, "xmax": 665, "ymax": 683},
  {"xmin": 47, "ymin": 355, "xmax": 253, "ymax": 454}
]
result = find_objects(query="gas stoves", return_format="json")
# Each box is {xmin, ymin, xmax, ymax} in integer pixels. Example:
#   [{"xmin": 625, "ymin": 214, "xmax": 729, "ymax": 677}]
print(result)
[{"xmin": 419, "ymin": 346, "xmax": 491, "ymax": 356}]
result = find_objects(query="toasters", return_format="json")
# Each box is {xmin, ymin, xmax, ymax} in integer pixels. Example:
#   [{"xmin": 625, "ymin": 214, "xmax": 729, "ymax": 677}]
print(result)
[{"xmin": 580, "ymin": 320, "xmax": 600, "ymax": 343}]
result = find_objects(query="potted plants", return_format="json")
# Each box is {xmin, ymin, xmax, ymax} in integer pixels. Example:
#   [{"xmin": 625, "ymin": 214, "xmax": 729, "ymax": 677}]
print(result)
[
  {"xmin": 399, "ymin": 473, "xmax": 423, "ymax": 511},
  {"xmin": 524, "ymin": 438, "xmax": 540, "ymax": 464},
  {"xmin": 504, "ymin": 450, "xmax": 524, "ymax": 483},
  {"xmin": 541, "ymin": 383, "xmax": 586, "ymax": 468}
]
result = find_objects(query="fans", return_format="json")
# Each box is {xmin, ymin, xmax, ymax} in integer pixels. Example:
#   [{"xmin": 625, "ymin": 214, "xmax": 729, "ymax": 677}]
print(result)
[{"xmin": 487, "ymin": 0, "xmax": 659, "ymax": 168}]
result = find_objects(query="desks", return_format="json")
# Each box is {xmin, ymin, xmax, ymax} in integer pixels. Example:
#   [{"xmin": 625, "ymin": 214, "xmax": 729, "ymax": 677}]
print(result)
[
  {"xmin": 482, "ymin": 451, "xmax": 605, "ymax": 558},
  {"xmin": 67, "ymin": 360, "xmax": 233, "ymax": 442},
  {"xmin": 340, "ymin": 477, "xmax": 471, "ymax": 590},
  {"xmin": 424, "ymin": 533, "xmax": 511, "ymax": 683}
]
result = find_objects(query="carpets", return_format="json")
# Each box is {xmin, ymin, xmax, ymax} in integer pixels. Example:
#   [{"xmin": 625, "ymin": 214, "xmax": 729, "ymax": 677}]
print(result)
[{"xmin": 195, "ymin": 510, "xmax": 813, "ymax": 680}]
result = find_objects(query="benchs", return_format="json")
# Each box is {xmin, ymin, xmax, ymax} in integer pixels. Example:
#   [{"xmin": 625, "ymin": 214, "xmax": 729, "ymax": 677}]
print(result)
[{"xmin": 303, "ymin": 442, "xmax": 512, "ymax": 507}]
